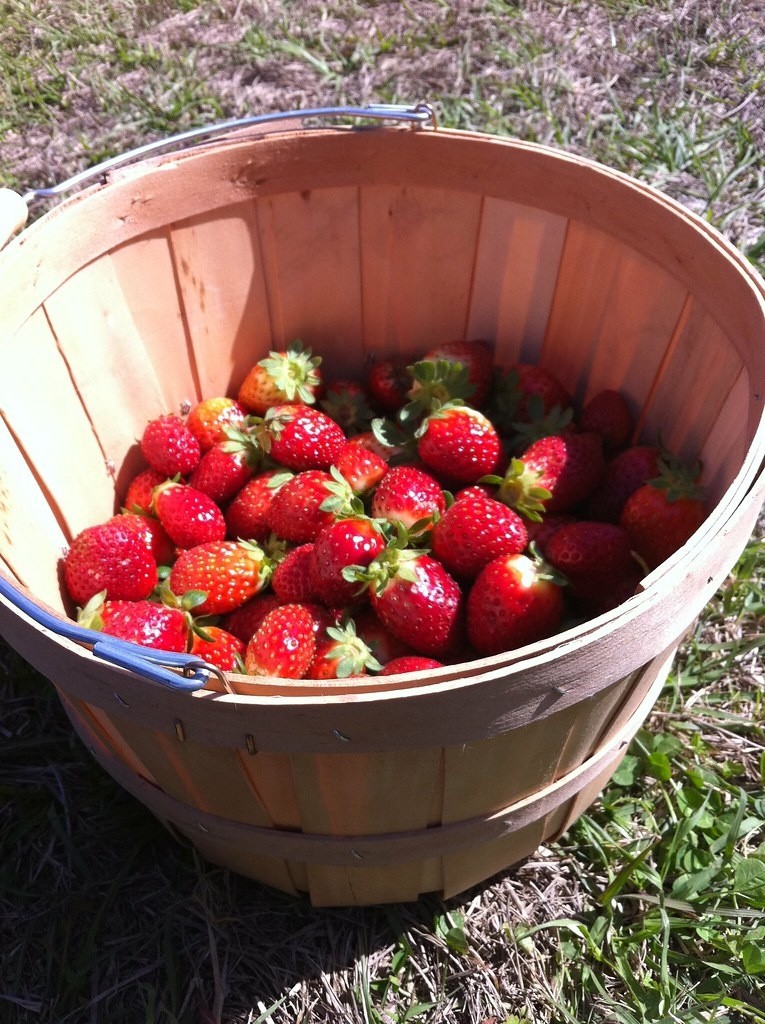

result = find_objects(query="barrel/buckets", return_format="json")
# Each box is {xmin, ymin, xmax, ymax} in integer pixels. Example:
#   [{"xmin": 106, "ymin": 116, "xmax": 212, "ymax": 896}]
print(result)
[{"xmin": 2, "ymin": 104, "xmax": 765, "ymax": 908}]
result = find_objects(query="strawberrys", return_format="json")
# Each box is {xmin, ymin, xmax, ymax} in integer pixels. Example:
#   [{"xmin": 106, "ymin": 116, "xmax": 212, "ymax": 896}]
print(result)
[{"xmin": 63, "ymin": 334, "xmax": 709, "ymax": 680}]
